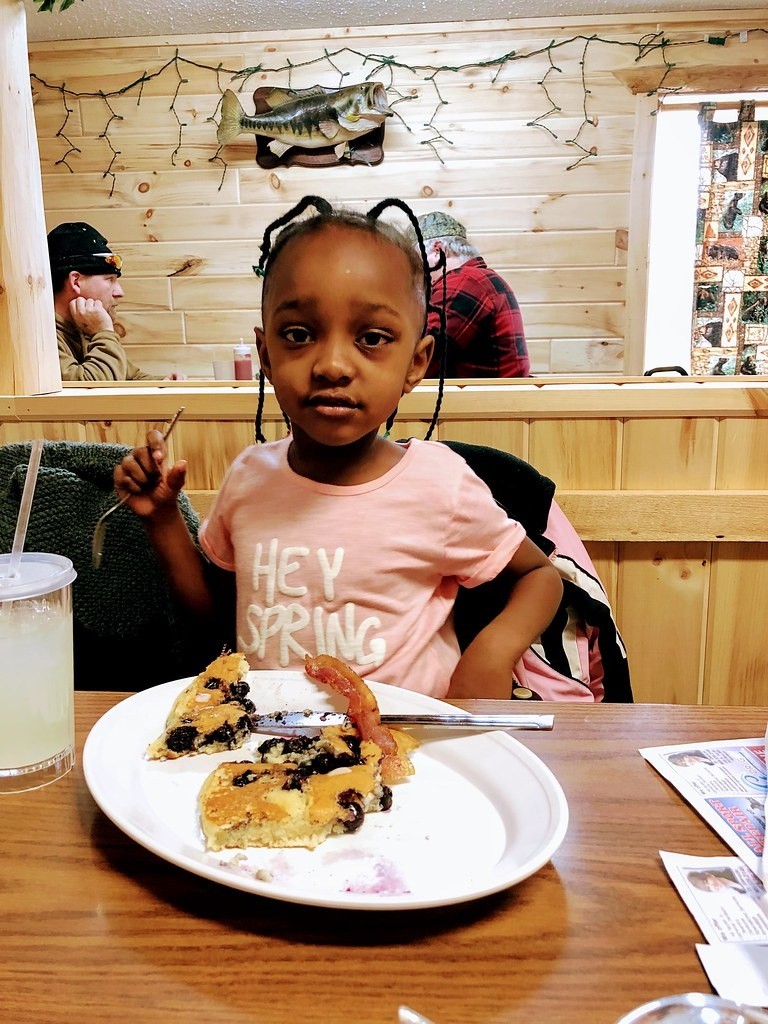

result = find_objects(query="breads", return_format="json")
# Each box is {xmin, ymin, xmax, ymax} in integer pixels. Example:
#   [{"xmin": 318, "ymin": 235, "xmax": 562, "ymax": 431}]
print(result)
[
  {"xmin": 196, "ymin": 753, "xmax": 394, "ymax": 851},
  {"xmin": 143, "ymin": 652, "xmax": 250, "ymax": 760},
  {"xmin": 257, "ymin": 724, "xmax": 419, "ymax": 782}
]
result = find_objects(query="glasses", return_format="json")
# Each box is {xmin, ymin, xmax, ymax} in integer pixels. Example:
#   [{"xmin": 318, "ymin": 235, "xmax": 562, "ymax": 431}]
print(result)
[{"xmin": 60, "ymin": 253, "xmax": 123, "ymax": 270}]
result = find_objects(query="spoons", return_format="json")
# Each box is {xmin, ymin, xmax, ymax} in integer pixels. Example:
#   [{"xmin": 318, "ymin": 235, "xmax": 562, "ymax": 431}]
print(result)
[{"xmin": 93, "ymin": 406, "xmax": 185, "ymax": 570}]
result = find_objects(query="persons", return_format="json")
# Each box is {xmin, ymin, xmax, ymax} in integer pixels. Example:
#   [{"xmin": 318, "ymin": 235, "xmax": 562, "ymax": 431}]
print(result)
[
  {"xmin": 404, "ymin": 212, "xmax": 530, "ymax": 378},
  {"xmin": 114, "ymin": 196, "xmax": 563, "ymax": 701},
  {"xmin": 48, "ymin": 223, "xmax": 187, "ymax": 381}
]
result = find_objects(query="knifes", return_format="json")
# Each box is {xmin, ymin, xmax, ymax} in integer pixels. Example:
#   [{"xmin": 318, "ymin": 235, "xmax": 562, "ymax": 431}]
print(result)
[{"xmin": 252, "ymin": 712, "xmax": 555, "ymax": 729}]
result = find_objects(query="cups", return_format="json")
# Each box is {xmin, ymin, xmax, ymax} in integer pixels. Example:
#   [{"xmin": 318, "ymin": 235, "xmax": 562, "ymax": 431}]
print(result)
[
  {"xmin": 213, "ymin": 361, "xmax": 233, "ymax": 379},
  {"xmin": 233, "ymin": 346, "xmax": 253, "ymax": 380},
  {"xmin": 0, "ymin": 552, "xmax": 78, "ymax": 795}
]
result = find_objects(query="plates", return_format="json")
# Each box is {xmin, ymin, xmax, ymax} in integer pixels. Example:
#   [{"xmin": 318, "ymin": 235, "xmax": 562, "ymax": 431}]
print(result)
[{"xmin": 83, "ymin": 670, "xmax": 569, "ymax": 911}]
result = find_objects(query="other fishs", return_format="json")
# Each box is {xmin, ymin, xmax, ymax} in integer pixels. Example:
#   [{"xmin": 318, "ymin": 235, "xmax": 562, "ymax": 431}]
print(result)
[{"xmin": 215, "ymin": 81, "xmax": 395, "ymax": 160}]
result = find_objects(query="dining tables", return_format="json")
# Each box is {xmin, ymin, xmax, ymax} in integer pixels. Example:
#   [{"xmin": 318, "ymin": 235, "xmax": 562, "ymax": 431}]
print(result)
[{"xmin": 0, "ymin": 689, "xmax": 768, "ymax": 1024}]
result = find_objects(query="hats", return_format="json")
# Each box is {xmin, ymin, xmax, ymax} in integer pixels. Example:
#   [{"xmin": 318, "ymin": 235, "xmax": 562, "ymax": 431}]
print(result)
[
  {"xmin": 405, "ymin": 212, "xmax": 468, "ymax": 238},
  {"xmin": 47, "ymin": 220, "xmax": 123, "ymax": 278}
]
